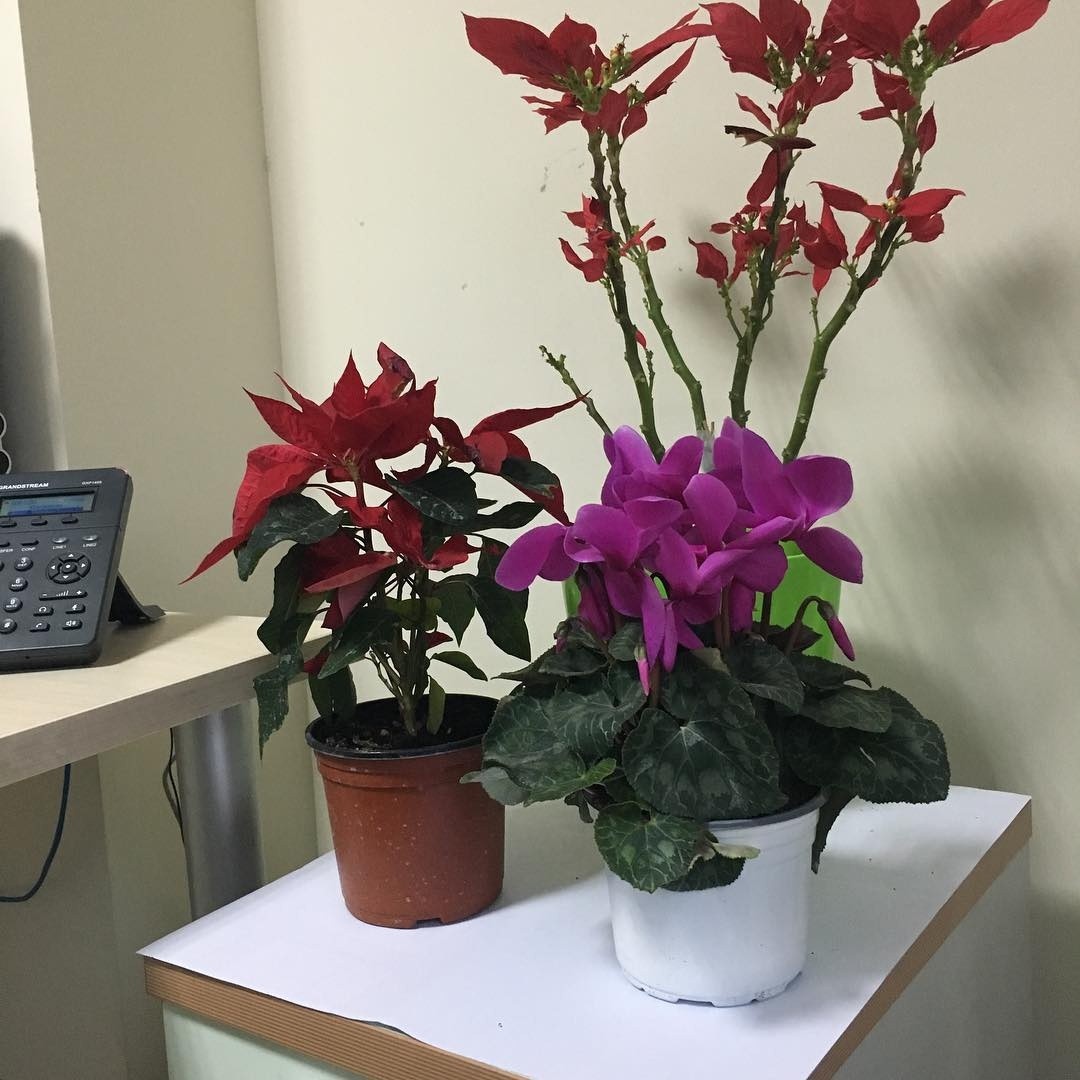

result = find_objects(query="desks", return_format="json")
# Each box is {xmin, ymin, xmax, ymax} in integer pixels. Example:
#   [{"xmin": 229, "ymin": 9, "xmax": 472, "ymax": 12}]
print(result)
[
  {"xmin": 0, "ymin": 607, "xmax": 333, "ymax": 923},
  {"xmin": 137, "ymin": 783, "xmax": 1033, "ymax": 1080}
]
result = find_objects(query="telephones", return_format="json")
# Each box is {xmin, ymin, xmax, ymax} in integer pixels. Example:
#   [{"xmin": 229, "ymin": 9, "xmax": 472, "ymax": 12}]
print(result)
[{"xmin": 0, "ymin": 468, "xmax": 166, "ymax": 674}]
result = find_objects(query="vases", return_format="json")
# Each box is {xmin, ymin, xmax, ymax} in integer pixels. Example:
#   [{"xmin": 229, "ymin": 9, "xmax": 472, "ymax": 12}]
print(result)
[
  {"xmin": 605, "ymin": 794, "xmax": 828, "ymax": 1008},
  {"xmin": 304, "ymin": 692, "xmax": 504, "ymax": 929},
  {"xmin": 562, "ymin": 527, "xmax": 842, "ymax": 664}
]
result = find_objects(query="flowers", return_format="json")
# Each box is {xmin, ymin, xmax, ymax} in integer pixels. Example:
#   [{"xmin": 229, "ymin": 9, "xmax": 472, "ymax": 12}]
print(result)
[
  {"xmin": 461, "ymin": 0, "xmax": 1049, "ymax": 466},
  {"xmin": 459, "ymin": 415, "xmax": 950, "ymax": 896},
  {"xmin": 175, "ymin": 341, "xmax": 592, "ymax": 767}
]
